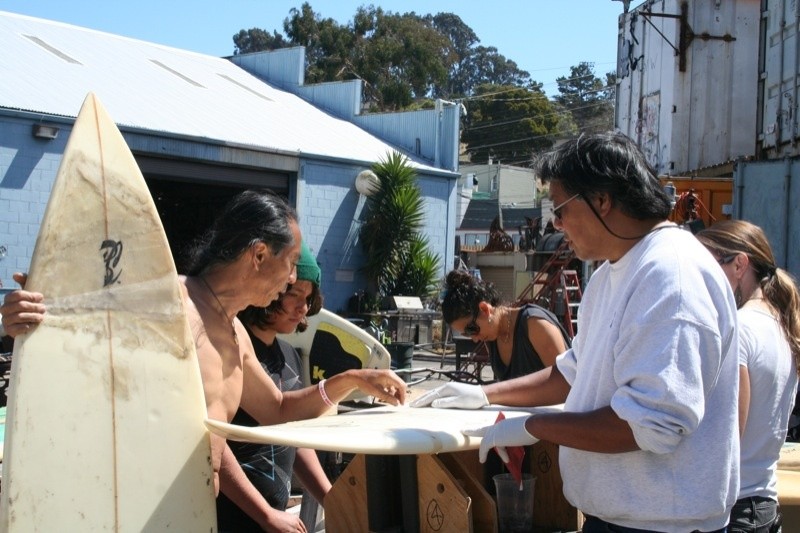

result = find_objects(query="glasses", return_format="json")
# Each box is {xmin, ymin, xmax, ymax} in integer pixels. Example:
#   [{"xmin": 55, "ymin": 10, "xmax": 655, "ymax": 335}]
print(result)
[
  {"xmin": 550, "ymin": 190, "xmax": 585, "ymax": 220},
  {"xmin": 461, "ymin": 309, "xmax": 480, "ymax": 338}
]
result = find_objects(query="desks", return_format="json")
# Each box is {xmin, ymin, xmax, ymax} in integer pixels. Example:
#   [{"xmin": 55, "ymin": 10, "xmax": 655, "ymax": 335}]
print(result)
[{"xmin": 203, "ymin": 402, "xmax": 565, "ymax": 532}]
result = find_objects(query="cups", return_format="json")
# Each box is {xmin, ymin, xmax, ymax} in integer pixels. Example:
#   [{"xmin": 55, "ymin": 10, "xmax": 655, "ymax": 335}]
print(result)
[{"xmin": 493, "ymin": 473, "xmax": 538, "ymax": 531}]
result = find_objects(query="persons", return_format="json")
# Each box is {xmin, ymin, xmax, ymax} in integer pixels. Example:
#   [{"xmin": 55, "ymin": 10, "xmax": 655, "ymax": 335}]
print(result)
[
  {"xmin": 0, "ymin": 189, "xmax": 409, "ymax": 533},
  {"xmin": 411, "ymin": 130, "xmax": 741, "ymax": 533},
  {"xmin": 443, "ymin": 270, "xmax": 579, "ymax": 532},
  {"xmin": 696, "ymin": 217, "xmax": 800, "ymax": 533},
  {"xmin": 208, "ymin": 229, "xmax": 333, "ymax": 533}
]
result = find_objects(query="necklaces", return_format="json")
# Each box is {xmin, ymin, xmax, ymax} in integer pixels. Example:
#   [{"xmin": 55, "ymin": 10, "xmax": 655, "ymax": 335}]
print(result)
[
  {"xmin": 500, "ymin": 307, "xmax": 511, "ymax": 344},
  {"xmin": 201, "ymin": 275, "xmax": 238, "ymax": 344}
]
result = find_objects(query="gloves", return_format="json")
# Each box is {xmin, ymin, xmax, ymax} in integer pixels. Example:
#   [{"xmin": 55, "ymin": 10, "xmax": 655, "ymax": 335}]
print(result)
[
  {"xmin": 462, "ymin": 414, "xmax": 542, "ymax": 464},
  {"xmin": 408, "ymin": 381, "xmax": 489, "ymax": 409}
]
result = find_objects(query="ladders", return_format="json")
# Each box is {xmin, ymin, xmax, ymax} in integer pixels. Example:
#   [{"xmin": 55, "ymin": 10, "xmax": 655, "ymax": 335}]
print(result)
[{"xmin": 550, "ymin": 269, "xmax": 583, "ymax": 340}]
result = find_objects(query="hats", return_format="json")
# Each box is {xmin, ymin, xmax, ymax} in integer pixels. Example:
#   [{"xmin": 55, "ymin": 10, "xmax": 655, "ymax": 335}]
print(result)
[{"xmin": 296, "ymin": 239, "xmax": 322, "ymax": 287}]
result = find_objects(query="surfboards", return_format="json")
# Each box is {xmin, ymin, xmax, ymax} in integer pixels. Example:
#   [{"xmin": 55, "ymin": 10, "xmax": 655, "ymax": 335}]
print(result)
[
  {"xmin": 202, "ymin": 401, "xmax": 565, "ymax": 456},
  {"xmin": 271, "ymin": 304, "xmax": 392, "ymax": 404},
  {"xmin": 0, "ymin": 91, "xmax": 220, "ymax": 532}
]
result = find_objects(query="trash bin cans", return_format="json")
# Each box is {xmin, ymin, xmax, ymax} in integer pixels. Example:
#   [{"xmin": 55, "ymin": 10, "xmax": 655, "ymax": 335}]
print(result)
[{"xmin": 381, "ymin": 341, "xmax": 415, "ymax": 386}]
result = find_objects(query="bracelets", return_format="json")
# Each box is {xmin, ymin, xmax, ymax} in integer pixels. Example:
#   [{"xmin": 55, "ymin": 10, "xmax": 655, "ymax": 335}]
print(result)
[{"xmin": 318, "ymin": 379, "xmax": 338, "ymax": 409}]
